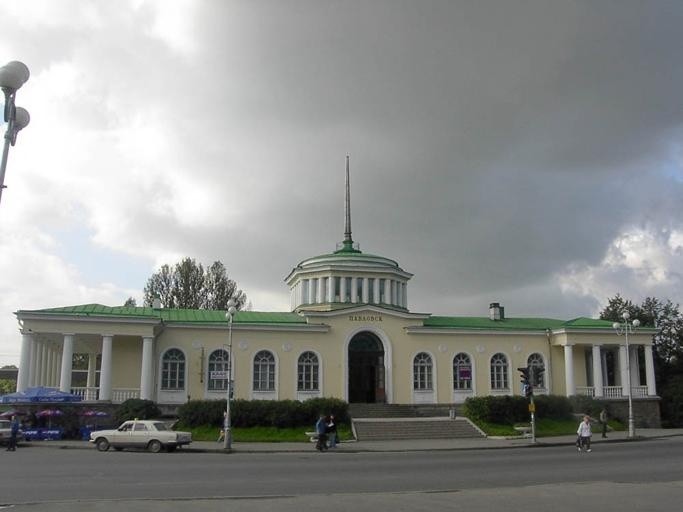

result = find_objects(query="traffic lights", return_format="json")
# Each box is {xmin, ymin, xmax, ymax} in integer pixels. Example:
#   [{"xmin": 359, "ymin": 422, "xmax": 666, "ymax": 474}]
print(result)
[
  {"xmin": 534, "ymin": 367, "xmax": 544, "ymax": 385},
  {"xmin": 517, "ymin": 367, "xmax": 529, "ymax": 384}
]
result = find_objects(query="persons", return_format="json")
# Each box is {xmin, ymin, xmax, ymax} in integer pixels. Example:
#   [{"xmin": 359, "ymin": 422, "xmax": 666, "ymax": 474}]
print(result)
[
  {"xmin": 314, "ymin": 412, "xmax": 329, "ymax": 451},
  {"xmin": 599, "ymin": 406, "xmax": 611, "ymax": 438},
  {"xmin": 325, "ymin": 413, "xmax": 337, "ymax": 448},
  {"xmin": 574, "ymin": 415, "xmax": 592, "ymax": 453},
  {"xmin": 4, "ymin": 415, "xmax": 19, "ymax": 451}
]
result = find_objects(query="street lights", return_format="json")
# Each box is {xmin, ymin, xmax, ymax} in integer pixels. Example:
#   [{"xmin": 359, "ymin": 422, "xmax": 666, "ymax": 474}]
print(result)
[
  {"xmin": 612, "ymin": 312, "xmax": 640, "ymax": 436},
  {"xmin": 222, "ymin": 300, "xmax": 236, "ymax": 452},
  {"xmin": 0, "ymin": 61, "xmax": 31, "ymax": 203}
]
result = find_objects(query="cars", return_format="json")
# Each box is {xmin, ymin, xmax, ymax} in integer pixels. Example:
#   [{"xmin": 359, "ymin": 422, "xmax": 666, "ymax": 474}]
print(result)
[
  {"xmin": 88, "ymin": 420, "xmax": 191, "ymax": 452},
  {"xmin": 0, "ymin": 419, "xmax": 23, "ymax": 445}
]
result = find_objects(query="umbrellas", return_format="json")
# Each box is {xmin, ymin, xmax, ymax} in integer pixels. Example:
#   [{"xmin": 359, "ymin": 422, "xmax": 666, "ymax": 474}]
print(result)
[
  {"xmin": 34, "ymin": 408, "xmax": 67, "ymax": 429},
  {"xmin": 0, "ymin": 409, "xmax": 26, "ymax": 416},
  {"xmin": 71, "ymin": 407, "xmax": 111, "ymax": 428}
]
